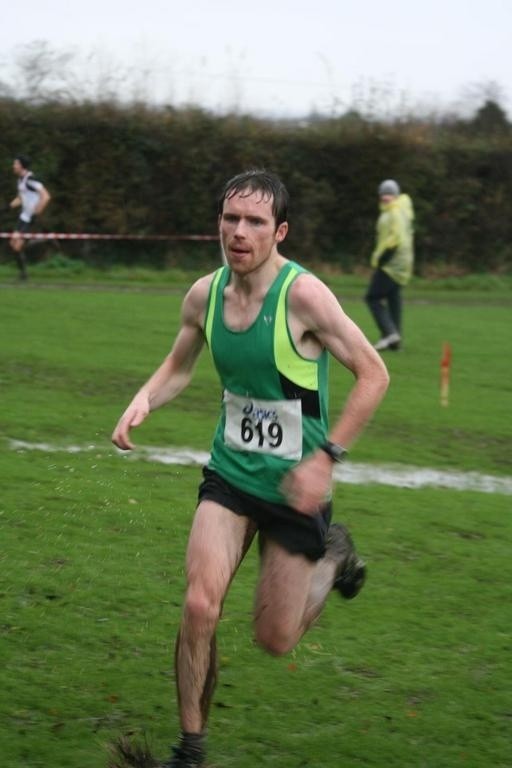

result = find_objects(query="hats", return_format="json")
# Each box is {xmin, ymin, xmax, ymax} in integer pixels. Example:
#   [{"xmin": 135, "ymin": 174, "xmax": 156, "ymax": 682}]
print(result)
[{"xmin": 378, "ymin": 179, "xmax": 399, "ymax": 196}]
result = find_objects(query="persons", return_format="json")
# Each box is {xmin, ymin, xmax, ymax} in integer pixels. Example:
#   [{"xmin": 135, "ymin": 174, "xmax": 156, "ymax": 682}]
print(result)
[
  {"xmin": 110, "ymin": 170, "xmax": 390, "ymax": 766},
  {"xmin": 1, "ymin": 153, "xmax": 61, "ymax": 281},
  {"xmin": 363, "ymin": 179, "xmax": 417, "ymax": 351}
]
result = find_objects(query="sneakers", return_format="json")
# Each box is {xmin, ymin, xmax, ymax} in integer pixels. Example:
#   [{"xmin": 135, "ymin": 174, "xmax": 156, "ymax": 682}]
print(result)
[
  {"xmin": 325, "ymin": 522, "xmax": 367, "ymax": 600},
  {"xmin": 374, "ymin": 331, "xmax": 402, "ymax": 350},
  {"xmin": 161, "ymin": 747, "xmax": 205, "ymax": 767}
]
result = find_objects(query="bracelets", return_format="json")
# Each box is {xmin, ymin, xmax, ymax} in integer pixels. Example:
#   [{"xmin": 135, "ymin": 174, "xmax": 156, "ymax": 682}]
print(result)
[{"xmin": 319, "ymin": 438, "xmax": 350, "ymax": 464}]
